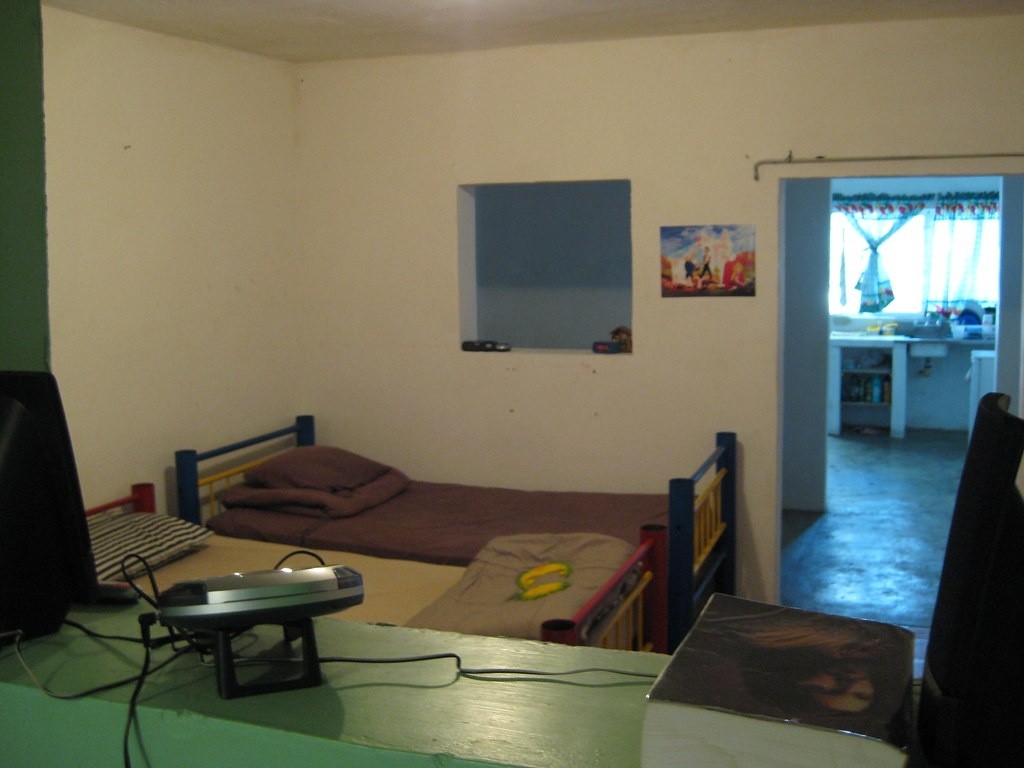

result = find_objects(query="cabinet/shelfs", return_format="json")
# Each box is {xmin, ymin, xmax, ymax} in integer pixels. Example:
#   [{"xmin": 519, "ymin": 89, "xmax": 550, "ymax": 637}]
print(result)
[{"xmin": 826, "ymin": 331, "xmax": 908, "ymax": 439}]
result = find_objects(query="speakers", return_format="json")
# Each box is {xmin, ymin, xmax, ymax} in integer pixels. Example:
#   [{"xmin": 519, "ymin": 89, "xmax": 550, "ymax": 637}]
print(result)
[{"xmin": 0, "ymin": 367, "xmax": 98, "ymax": 645}]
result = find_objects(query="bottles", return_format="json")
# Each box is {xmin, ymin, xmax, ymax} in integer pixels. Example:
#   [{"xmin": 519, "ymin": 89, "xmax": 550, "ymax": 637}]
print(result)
[
  {"xmin": 982, "ymin": 308, "xmax": 995, "ymax": 337},
  {"xmin": 883, "ymin": 375, "xmax": 891, "ymax": 401},
  {"xmin": 872, "ymin": 376, "xmax": 882, "ymax": 402},
  {"xmin": 866, "ymin": 378, "xmax": 872, "ymax": 401}
]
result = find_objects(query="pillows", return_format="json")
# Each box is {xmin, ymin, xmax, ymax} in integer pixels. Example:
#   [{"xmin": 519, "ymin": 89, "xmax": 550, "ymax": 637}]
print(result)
[
  {"xmin": 242, "ymin": 446, "xmax": 390, "ymax": 491},
  {"xmin": 86, "ymin": 506, "xmax": 213, "ymax": 585}
]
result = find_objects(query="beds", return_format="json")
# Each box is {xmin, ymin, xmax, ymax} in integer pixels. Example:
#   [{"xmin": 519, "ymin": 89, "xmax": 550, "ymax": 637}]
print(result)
[
  {"xmin": 175, "ymin": 416, "xmax": 736, "ymax": 647},
  {"xmin": 86, "ymin": 483, "xmax": 672, "ymax": 652}
]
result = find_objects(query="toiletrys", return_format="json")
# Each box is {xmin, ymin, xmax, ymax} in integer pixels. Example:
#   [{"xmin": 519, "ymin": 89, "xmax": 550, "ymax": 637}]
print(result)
[
  {"xmin": 981, "ymin": 314, "xmax": 992, "ymax": 339},
  {"xmin": 849, "ymin": 374, "xmax": 889, "ymax": 403}
]
